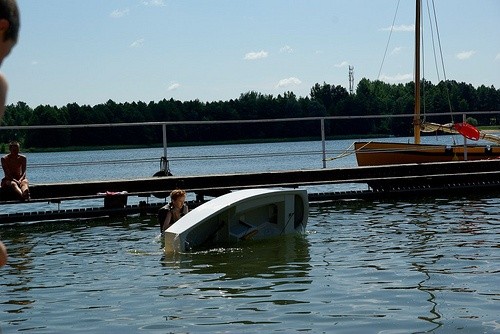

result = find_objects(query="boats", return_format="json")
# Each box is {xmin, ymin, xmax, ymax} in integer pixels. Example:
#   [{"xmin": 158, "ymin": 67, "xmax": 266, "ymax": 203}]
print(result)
[
  {"xmin": 355, "ymin": 0, "xmax": 500, "ymax": 191},
  {"xmin": 164, "ymin": 187, "xmax": 309, "ymax": 255}
]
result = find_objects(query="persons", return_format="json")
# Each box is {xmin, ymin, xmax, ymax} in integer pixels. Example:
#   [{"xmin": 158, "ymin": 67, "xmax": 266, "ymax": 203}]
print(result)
[
  {"xmin": 2, "ymin": 142, "xmax": 32, "ymax": 202},
  {"xmin": 159, "ymin": 190, "xmax": 189, "ymax": 234}
]
